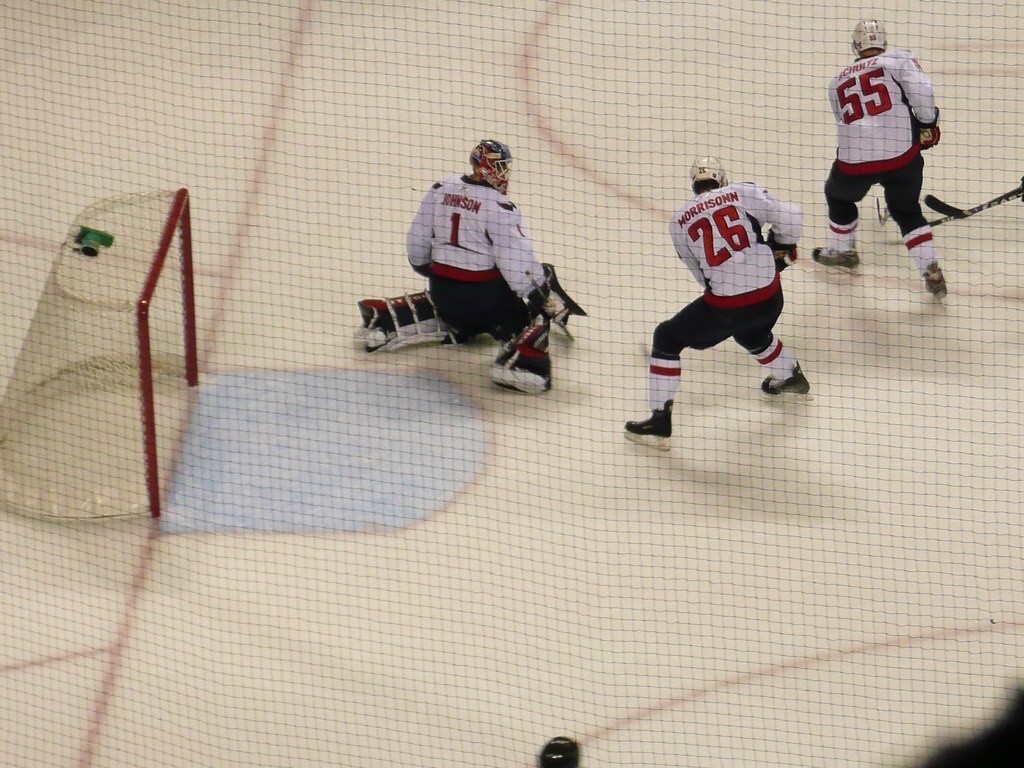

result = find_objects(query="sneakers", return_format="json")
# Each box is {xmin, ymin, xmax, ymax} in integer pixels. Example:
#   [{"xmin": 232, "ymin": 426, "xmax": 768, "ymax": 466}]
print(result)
[
  {"xmin": 813, "ymin": 246, "xmax": 861, "ymax": 275},
  {"xmin": 922, "ymin": 261, "xmax": 948, "ymax": 301}
]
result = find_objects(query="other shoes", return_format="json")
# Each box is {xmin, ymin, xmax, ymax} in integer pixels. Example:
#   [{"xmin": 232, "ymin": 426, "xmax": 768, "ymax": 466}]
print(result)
[
  {"xmin": 488, "ymin": 364, "xmax": 546, "ymax": 395},
  {"xmin": 347, "ymin": 330, "xmax": 386, "ymax": 348}
]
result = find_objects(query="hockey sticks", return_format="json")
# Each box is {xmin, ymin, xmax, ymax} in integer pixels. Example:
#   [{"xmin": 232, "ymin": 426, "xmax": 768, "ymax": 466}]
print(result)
[
  {"xmin": 526, "ymin": 270, "xmax": 574, "ymax": 342},
  {"xmin": 924, "ymin": 187, "xmax": 1024, "ymax": 227},
  {"xmin": 875, "ymin": 131, "xmax": 932, "ymax": 225}
]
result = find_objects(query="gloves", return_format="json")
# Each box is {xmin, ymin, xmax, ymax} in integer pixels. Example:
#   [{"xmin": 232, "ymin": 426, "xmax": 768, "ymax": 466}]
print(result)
[{"xmin": 919, "ymin": 106, "xmax": 942, "ymax": 147}]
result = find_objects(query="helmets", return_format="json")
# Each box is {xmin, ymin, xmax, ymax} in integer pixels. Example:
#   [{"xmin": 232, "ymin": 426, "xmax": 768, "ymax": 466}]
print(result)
[
  {"xmin": 470, "ymin": 138, "xmax": 513, "ymax": 195},
  {"xmin": 852, "ymin": 19, "xmax": 887, "ymax": 52},
  {"xmin": 689, "ymin": 155, "xmax": 726, "ymax": 190}
]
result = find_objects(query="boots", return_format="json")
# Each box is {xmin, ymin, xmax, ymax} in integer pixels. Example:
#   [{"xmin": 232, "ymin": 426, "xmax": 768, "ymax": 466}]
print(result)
[
  {"xmin": 760, "ymin": 360, "xmax": 814, "ymax": 400},
  {"xmin": 624, "ymin": 399, "xmax": 675, "ymax": 451}
]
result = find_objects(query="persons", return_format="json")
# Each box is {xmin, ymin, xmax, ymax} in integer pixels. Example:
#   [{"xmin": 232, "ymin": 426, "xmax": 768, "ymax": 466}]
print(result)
[
  {"xmin": 623, "ymin": 155, "xmax": 809, "ymax": 451},
  {"xmin": 360, "ymin": 139, "xmax": 553, "ymax": 393},
  {"xmin": 813, "ymin": 18, "xmax": 949, "ymax": 306}
]
